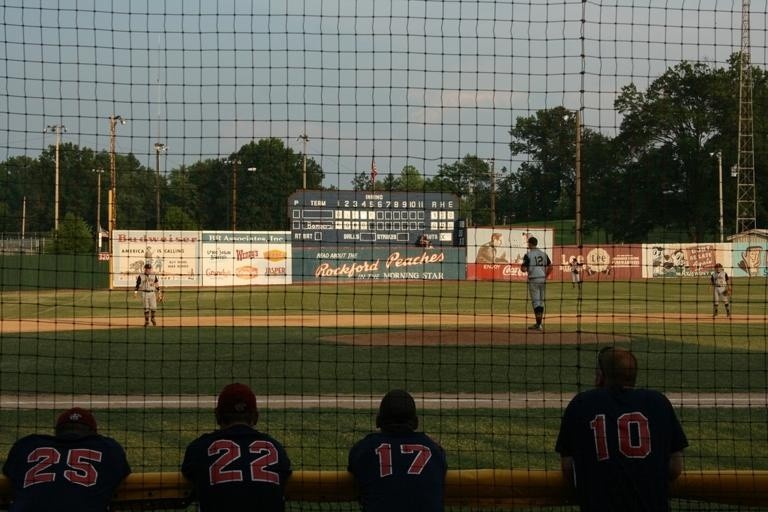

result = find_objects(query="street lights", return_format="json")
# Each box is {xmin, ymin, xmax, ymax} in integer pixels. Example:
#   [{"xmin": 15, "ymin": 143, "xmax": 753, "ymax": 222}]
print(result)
[
  {"xmin": 562, "ymin": 109, "xmax": 581, "ymax": 245},
  {"xmin": 486, "ymin": 156, "xmax": 496, "ymax": 229},
  {"xmin": 224, "ymin": 159, "xmax": 242, "ymax": 231},
  {"xmin": 297, "ymin": 133, "xmax": 310, "ymax": 189},
  {"xmin": 716, "ymin": 151, "xmax": 724, "ymax": 243},
  {"xmin": 109, "ymin": 115, "xmax": 126, "ymax": 230},
  {"xmin": 154, "ymin": 142, "xmax": 168, "ymax": 230},
  {"xmin": 43, "ymin": 124, "xmax": 67, "ymax": 230},
  {"xmin": 92, "ymin": 167, "xmax": 105, "ymax": 253}
]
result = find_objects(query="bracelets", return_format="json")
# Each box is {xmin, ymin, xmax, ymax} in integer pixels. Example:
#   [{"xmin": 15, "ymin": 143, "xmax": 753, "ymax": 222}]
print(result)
[
  {"xmin": 156, "ymin": 287, "xmax": 160, "ymax": 290},
  {"xmin": 728, "ymin": 288, "xmax": 732, "ymax": 291}
]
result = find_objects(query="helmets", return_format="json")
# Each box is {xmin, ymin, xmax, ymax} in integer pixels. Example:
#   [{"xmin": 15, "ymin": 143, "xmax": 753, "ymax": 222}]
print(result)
[
  {"xmin": 714, "ymin": 263, "xmax": 722, "ymax": 268},
  {"xmin": 144, "ymin": 264, "xmax": 152, "ymax": 269}
]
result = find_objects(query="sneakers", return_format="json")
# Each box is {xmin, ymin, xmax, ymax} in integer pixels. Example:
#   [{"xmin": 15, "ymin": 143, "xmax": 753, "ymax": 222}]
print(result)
[
  {"xmin": 143, "ymin": 319, "xmax": 157, "ymax": 327},
  {"xmin": 527, "ymin": 323, "xmax": 543, "ymax": 330}
]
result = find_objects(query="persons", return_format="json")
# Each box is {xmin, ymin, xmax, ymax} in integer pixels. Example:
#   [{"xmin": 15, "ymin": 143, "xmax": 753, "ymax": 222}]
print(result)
[
  {"xmin": 513, "ymin": 232, "xmax": 533, "ymax": 264},
  {"xmin": 475, "ymin": 233, "xmax": 507, "ymax": 264},
  {"xmin": 568, "ymin": 258, "xmax": 584, "ymax": 288},
  {"xmin": 133, "ymin": 263, "xmax": 161, "ymax": 327},
  {"xmin": 181, "ymin": 381, "xmax": 294, "ymax": 512},
  {"xmin": 345, "ymin": 390, "xmax": 448, "ymax": 511},
  {"xmin": 709, "ymin": 263, "xmax": 732, "ymax": 320},
  {"xmin": 520, "ymin": 237, "xmax": 553, "ymax": 329},
  {"xmin": 554, "ymin": 346, "xmax": 689, "ymax": 511},
  {"xmin": 415, "ymin": 235, "xmax": 428, "ymax": 246},
  {"xmin": 2, "ymin": 407, "xmax": 132, "ymax": 511}
]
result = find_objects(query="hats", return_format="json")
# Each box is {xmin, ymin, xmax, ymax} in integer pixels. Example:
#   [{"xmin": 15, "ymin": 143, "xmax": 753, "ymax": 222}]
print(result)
[
  {"xmin": 214, "ymin": 381, "xmax": 259, "ymax": 414},
  {"xmin": 379, "ymin": 387, "xmax": 417, "ymax": 418},
  {"xmin": 56, "ymin": 407, "xmax": 96, "ymax": 427}
]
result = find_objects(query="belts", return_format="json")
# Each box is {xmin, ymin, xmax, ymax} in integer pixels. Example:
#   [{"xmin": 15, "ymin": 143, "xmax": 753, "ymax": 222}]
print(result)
[{"xmin": 142, "ymin": 289, "xmax": 154, "ymax": 293}]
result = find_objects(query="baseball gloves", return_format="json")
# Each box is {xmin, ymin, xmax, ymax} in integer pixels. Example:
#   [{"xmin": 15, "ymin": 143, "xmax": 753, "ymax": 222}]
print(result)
[
  {"xmin": 723, "ymin": 289, "xmax": 733, "ymax": 296},
  {"xmin": 574, "ymin": 269, "xmax": 577, "ymax": 273},
  {"xmin": 157, "ymin": 291, "xmax": 164, "ymax": 301}
]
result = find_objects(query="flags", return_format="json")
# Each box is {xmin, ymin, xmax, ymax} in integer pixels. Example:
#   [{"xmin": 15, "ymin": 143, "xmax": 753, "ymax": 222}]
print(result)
[{"xmin": 370, "ymin": 158, "xmax": 377, "ymax": 179}]
